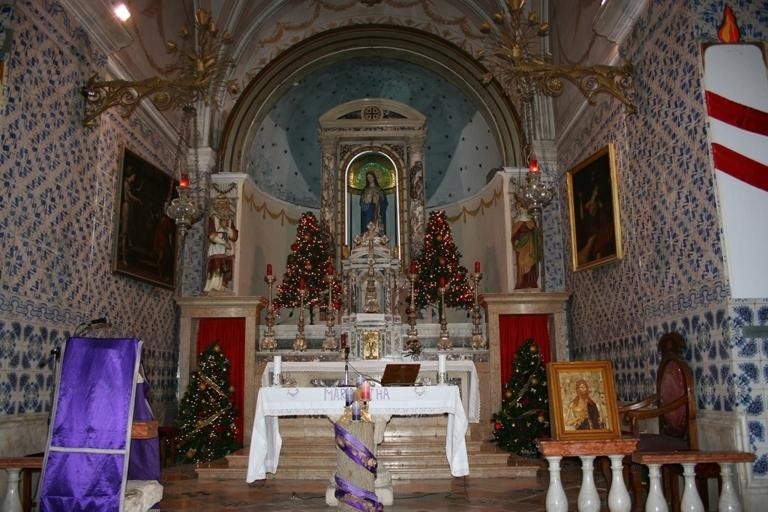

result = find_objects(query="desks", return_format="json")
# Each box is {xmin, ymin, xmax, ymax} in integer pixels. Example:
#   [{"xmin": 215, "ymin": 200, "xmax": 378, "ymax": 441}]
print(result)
[
  {"xmin": 246, "ymin": 384, "xmax": 469, "ymax": 482},
  {"xmin": 1, "ymin": 458, "xmax": 47, "ymax": 512},
  {"xmin": 261, "ymin": 359, "xmax": 480, "ymax": 424}
]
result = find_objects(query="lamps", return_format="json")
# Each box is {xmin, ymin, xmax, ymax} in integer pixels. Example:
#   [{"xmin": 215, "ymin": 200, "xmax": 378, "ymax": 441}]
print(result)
[{"xmin": 180, "ymin": 174, "xmax": 190, "ymax": 187}]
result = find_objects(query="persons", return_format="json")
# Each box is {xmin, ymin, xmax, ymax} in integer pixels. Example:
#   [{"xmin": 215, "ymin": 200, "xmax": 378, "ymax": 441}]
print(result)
[
  {"xmin": 205, "ymin": 195, "xmax": 238, "ymax": 294},
  {"xmin": 151, "ymin": 206, "xmax": 172, "ymax": 266},
  {"xmin": 121, "ymin": 165, "xmax": 144, "ymax": 267},
  {"xmin": 513, "ymin": 203, "xmax": 536, "ymax": 289},
  {"xmin": 566, "ymin": 381, "xmax": 602, "ymax": 430},
  {"xmin": 578, "ymin": 182, "xmax": 606, "ymax": 264},
  {"xmin": 360, "ymin": 171, "xmax": 388, "ymax": 237}
]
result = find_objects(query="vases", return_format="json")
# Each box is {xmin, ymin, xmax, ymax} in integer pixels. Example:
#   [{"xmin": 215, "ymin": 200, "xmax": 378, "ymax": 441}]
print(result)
[{"xmin": 0, "ymin": 469, "xmax": 24, "ymax": 512}]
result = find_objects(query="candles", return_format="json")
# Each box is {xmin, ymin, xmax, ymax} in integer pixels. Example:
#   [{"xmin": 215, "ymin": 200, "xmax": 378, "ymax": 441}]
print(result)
[
  {"xmin": 327, "ymin": 262, "xmax": 334, "ymax": 275},
  {"xmin": 341, "ymin": 334, "xmax": 347, "ymax": 350},
  {"xmin": 299, "ymin": 277, "xmax": 304, "ymax": 289},
  {"xmin": 346, "ymin": 386, "xmax": 354, "ymax": 405},
  {"xmin": 409, "ymin": 258, "xmax": 415, "ymax": 274},
  {"xmin": 474, "ymin": 262, "xmax": 480, "ymax": 273},
  {"xmin": 530, "ymin": 159, "xmax": 538, "ymax": 172},
  {"xmin": 266, "ymin": 264, "xmax": 272, "ymax": 274},
  {"xmin": 362, "ymin": 381, "xmax": 370, "ymax": 399},
  {"xmin": 438, "ymin": 275, "xmax": 445, "ymax": 287},
  {"xmin": 352, "ymin": 401, "xmax": 360, "ymax": 420},
  {"xmin": 356, "ymin": 374, "xmax": 364, "ymax": 389}
]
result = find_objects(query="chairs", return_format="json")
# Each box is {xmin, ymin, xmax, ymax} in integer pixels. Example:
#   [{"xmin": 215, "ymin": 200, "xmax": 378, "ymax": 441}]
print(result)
[{"xmin": 619, "ymin": 332, "xmax": 698, "ymax": 511}]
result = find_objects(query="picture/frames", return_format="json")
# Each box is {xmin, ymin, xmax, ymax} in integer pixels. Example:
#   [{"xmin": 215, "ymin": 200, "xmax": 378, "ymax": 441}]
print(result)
[
  {"xmin": 547, "ymin": 360, "xmax": 621, "ymax": 440},
  {"xmin": 566, "ymin": 142, "xmax": 623, "ymax": 274},
  {"xmin": 112, "ymin": 142, "xmax": 180, "ymax": 289}
]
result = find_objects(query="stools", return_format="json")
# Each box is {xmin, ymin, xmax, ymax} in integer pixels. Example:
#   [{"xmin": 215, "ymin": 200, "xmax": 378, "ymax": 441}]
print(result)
[{"xmin": 157, "ymin": 427, "xmax": 180, "ymax": 469}]
